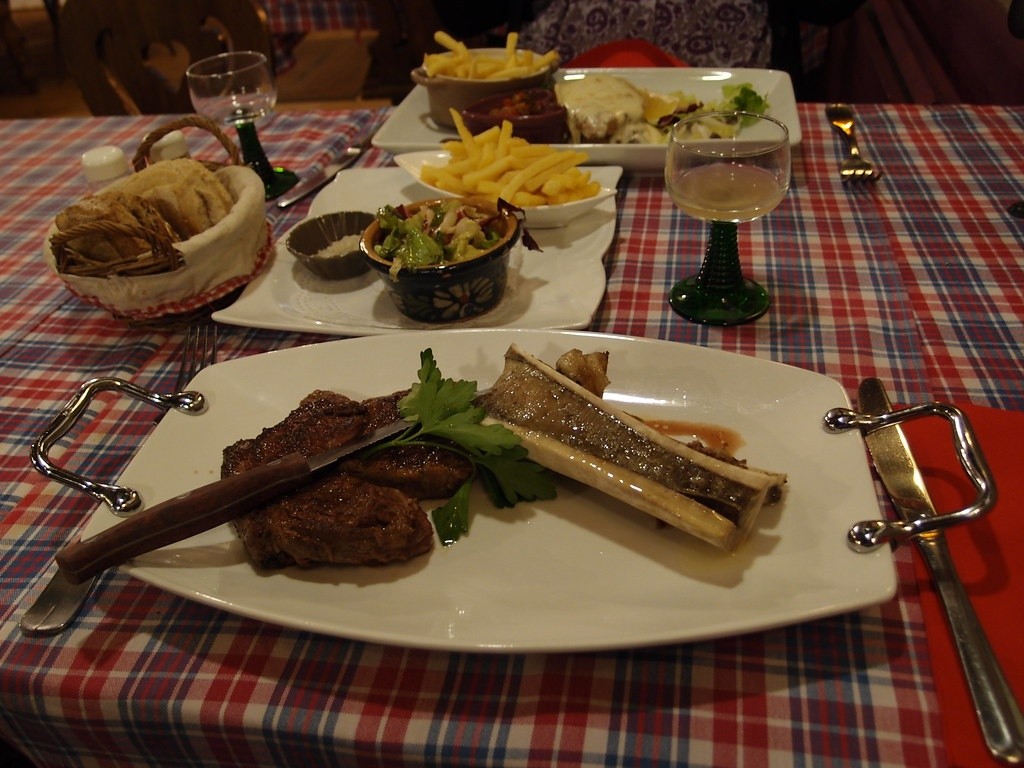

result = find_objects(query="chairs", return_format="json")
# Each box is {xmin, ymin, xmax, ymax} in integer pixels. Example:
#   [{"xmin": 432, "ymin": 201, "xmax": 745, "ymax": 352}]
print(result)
[{"xmin": 52, "ymin": 0, "xmax": 278, "ymax": 112}]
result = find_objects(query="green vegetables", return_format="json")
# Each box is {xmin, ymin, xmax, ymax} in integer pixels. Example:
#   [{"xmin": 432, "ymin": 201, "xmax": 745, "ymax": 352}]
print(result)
[{"xmin": 365, "ymin": 347, "xmax": 561, "ymax": 549}]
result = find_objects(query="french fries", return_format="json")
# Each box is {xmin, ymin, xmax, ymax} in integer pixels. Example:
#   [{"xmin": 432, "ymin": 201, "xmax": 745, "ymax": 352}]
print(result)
[
  {"xmin": 421, "ymin": 109, "xmax": 602, "ymax": 210},
  {"xmin": 421, "ymin": 29, "xmax": 560, "ymax": 83}
]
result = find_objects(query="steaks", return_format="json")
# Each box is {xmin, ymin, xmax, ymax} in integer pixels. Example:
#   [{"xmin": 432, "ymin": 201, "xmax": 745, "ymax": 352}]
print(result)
[{"xmin": 217, "ymin": 388, "xmax": 471, "ymax": 576}]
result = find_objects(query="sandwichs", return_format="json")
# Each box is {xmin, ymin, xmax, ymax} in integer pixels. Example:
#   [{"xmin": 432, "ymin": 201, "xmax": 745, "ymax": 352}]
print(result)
[{"xmin": 54, "ymin": 156, "xmax": 236, "ymax": 261}]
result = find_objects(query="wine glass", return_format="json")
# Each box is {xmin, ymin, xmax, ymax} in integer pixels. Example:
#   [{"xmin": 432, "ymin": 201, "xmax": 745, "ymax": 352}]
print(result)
[
  {"xmin": 661, "ymin": 108, "xmax": 793, "ymax": 324},
  {"xmin": 185, "ymin": 49, "xmax": 298, "ymax": 202}
]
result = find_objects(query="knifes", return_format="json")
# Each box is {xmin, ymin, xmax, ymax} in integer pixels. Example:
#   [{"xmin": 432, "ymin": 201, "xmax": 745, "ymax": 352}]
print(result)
[
  {"xmin": 855, "ymin": 375, "xmax": 1024, "ymax": 765},
  {"xmin": 275, "ymin": 114, "xmax": 389, "ymax": 207},
  {"xmin": 55, "ymin": 385, "xmax": 491, "ymax": 587}
]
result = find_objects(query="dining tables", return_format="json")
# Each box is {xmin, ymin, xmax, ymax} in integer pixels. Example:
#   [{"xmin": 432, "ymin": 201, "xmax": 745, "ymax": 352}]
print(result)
[{"xmin": 0, "ymin": 98, "xmax": 1024, "ymax": 768}]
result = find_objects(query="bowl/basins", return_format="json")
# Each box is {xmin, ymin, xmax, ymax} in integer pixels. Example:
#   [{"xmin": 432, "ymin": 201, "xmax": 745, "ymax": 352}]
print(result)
[
  {"xmin": 357, "ymin": 194, "xmax": 521, "ymax": 322},
  {"xmin": 414, "ymin": 46, "xmax": 554, "ymax": 125},
  {"xmin": 287, "ymin": 209, "xmax": 374, "ymax": 279},
  {"xmin": 460, "ymin": 90, "xmax": 569, "ymax": 147}
]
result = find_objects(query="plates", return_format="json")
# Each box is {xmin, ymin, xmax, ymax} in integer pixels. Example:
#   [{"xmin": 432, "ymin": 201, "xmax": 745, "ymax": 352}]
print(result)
[
  {"xmin": 211, "ymin": 162, "xmax": 628, "ymax": 335},
  {"xmin": 393, "ymin": 150, "xmax": 621, "ymax": 228},
  {"xmin": 371, "ymin": 65, "xmax": 801, "ymax": 169},
  {"xmin": 32, "ymin": 325, "xmax": 995, "ymax": 656}
]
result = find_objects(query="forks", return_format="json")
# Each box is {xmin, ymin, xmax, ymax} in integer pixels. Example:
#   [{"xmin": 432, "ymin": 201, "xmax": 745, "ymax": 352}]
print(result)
[
  {"xmin": 18, "ymin": 325, "xmax": 213, "ymax": 636},
  {"xmin": 825, "ymin": 104, "xmax": 884, "ymax": 186}
]
